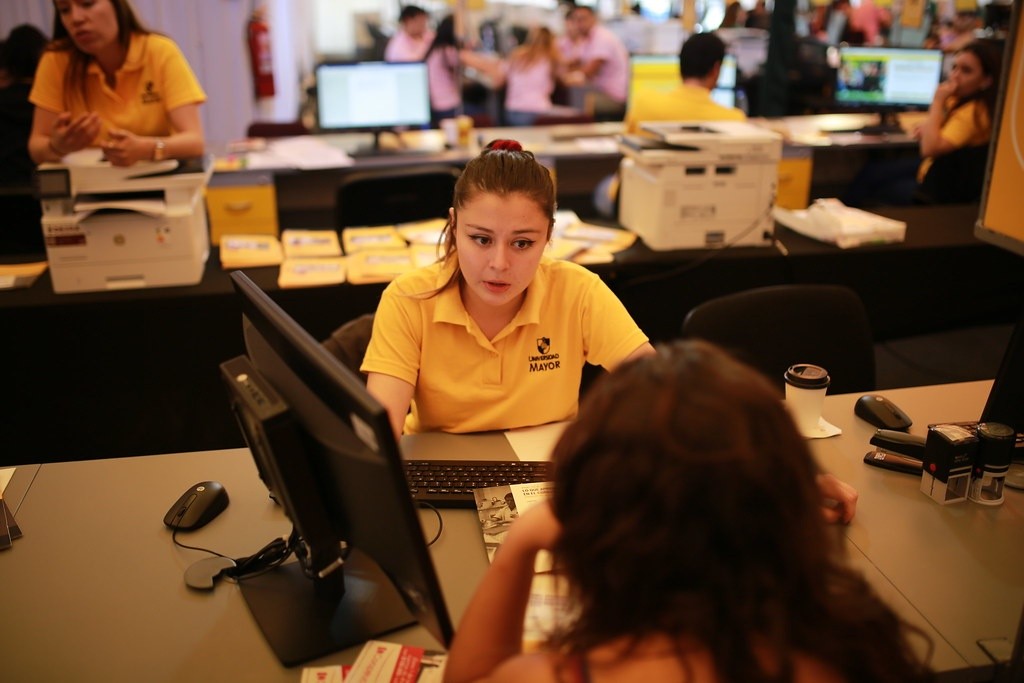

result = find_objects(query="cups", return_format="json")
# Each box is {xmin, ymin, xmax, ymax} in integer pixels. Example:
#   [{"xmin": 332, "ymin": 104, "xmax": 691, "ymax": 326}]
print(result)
[
  {"xmin": 441, "ymin": 118, "xmax": 457, "ymax": 145},
  {"xmin": 783, "ymin": 363, "xmax": 830, "ymax": 431}
]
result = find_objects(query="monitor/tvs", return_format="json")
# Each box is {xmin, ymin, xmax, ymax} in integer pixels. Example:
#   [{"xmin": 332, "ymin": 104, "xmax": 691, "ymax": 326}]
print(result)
[
  {"xmin": 833, "ymin": 45, "xmax": 945, "ymax": 132},
  {"xmin": 974, "ymin": 0, "xmax": 1023, "ymax": 426},
  {"xmin": 624, "ymin": 51, "xmax": 739, "ymax": 117},
  {"xmin": 314, "ymin": 60, "xmax": 433, "ymax": 157},
  {"xmin": 218, "ymin": 269, "xmax": 456, "ymax": 669}
]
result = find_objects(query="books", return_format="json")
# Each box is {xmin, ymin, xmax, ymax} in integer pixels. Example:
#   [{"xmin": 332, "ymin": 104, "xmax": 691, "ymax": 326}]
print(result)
[
  {"xmin": 617, "ymin": 119, "xmax": 783, "ymax": 164},
  {"xmin": 300, "ymin": 640, "xmax": 446, "ymax": 683},
  {"xmin": 0, "ymin": 488, "xmax": 23, "ymax": 551},
  {"xmin": 220, "ymin": 211, "xmax": 638, "ymax": 289},
  {"xmin": 0, "ymin": 261, "xmax": 48, "ymax": 289},
  {"xmin": 72, "ymin": 189, "xmax": 166, "ymax": 212}
]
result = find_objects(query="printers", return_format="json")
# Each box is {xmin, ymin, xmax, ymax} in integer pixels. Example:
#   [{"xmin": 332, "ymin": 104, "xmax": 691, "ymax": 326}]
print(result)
[
  {"xmin": 37, "ymin": 145, "xmax": 214, "ymax": 293},
  {"xmin": 616, "ymin": 119, "xmax": 781, "ymax": 250}
]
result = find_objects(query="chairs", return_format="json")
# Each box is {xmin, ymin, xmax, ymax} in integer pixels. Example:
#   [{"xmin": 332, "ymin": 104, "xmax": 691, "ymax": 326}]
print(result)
[
  {"xmin": 335, "ymin": 160, "xmax": 462, "ymax": 302},
  {"xmin": 674, "ymin": 281, "xmax": 878, "ymax": 398}
]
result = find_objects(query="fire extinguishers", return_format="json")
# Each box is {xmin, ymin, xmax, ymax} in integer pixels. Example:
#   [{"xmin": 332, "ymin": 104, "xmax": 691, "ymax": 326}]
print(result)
[{"xmin": 247, "ymin": 3, "xmax": 275, "ymax": 99}]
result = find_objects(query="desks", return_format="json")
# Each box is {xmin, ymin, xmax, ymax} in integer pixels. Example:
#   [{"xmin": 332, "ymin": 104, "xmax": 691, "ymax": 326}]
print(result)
[{"xmin": 1, "ymin": 123, "xmax": 1023, "ymax": 682}]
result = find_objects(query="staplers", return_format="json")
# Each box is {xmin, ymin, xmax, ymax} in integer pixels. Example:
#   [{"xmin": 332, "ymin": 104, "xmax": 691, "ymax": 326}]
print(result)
[{"xmin": 863, "ymin": 429, "xmax": 928, "ymax": 477}]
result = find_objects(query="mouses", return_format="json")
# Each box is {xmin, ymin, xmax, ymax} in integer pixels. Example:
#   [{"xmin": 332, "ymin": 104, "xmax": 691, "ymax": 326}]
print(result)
[
  {"xmin": 854, "ymin": 394, "xmax": 913, "ymax": 430},
  {"xmin": 162, "ymin": 481, "xmax": 228, "ymax": 530}
]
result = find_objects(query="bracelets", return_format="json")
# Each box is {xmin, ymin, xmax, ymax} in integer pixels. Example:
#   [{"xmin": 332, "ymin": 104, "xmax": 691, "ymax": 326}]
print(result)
[
  {"xmin": 151, "ymin": 137, "xmax": 165, "ymax": 160},
  {"xmin": 48, "ymin": 142, "xmax": 67, "ymax": 158}
]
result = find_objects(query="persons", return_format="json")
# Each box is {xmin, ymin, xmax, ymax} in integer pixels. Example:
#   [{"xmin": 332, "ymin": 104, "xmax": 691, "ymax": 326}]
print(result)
[
  {"xmin": 720, "ymin": 0, "xmax": 773, "ymax": 30},
  {"xmin": 912, "ymin": 44, "xmax": 1000, "ymax": 182},
  {"xmin": 359, "ymin": 139, "xmax": 858, "ymax": 526},
  {"xmin": 384, "ymin": 6, "xmax": 437, "ymax": 62},
  {"xmin": 440, "ymin": 341, "xmax": 941, "ymax": 683},
  {"xmin": 491, "ymin": 492, "xmax": 519, "ymax": 522},
  {"xmin": 810, "ymin": 1, "xmax": 983, "ymax": 52},
  {"xmin": 555, "ymin": 6, "xmax": 631, "ymax": 122},
  {"xmin": 624, "ymin": 32, "xmax": 746, "ymax": 140},
  {"xmin": 0, "ymin": 24, "xmax": 50, "ymax": 186},
  {"xmin": 27, "ymin": 0, "xmax": 208, "ymax": 168},
  {"xmin": 422, "ymin": 12, "xmax": 499, "ymax": 130},
  {"xmin": 499, "ymin": 25, "xmax": 586, "ymax": 125}
]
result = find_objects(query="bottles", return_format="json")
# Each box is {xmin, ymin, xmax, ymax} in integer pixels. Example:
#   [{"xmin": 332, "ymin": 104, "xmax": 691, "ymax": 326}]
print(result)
[{"xmin": 967, "ymin": 422, "xmax": 1016, "ymax": 505}]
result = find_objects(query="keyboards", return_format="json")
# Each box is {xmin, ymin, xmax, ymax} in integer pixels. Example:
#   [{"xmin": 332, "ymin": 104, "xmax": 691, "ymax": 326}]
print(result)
[
  {"xmin": 403, "ymin": 459, "xmax": 554, "ymax": 509},
  {"xmin": 927, "ymin": 420, "xmax": 1024, "ymax": 453}
]
